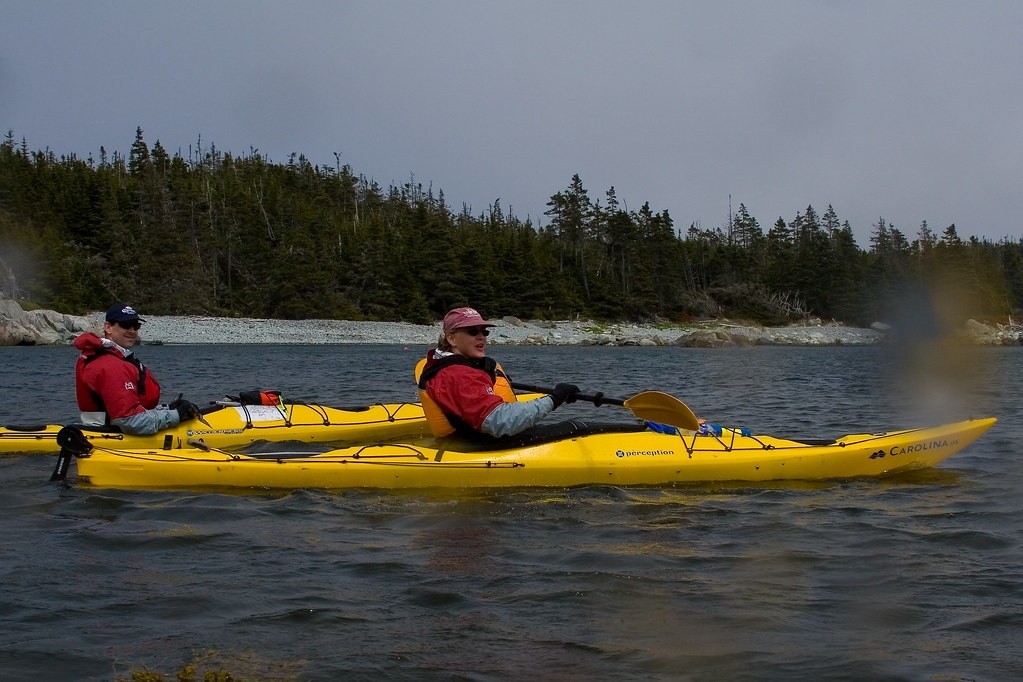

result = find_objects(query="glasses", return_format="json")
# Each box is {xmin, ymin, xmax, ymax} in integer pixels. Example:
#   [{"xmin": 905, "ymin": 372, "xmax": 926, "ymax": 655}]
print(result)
[
  {"xmin": 107, "ymin": 320, "xmax": 142, "ymax": 331},
  {"xmin": 449, "ymin": 326, "xmax": 490, "ymax": 336}
]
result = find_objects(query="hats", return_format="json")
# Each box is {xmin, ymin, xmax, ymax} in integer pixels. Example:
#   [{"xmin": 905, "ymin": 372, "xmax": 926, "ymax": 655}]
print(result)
[
  {"xmin": 106, "ymin": 304, "xmax": 147, "ymax": 322},
  {"xmin": 443, "ymin": 307, "xmax": 497, "ymax": 333}
]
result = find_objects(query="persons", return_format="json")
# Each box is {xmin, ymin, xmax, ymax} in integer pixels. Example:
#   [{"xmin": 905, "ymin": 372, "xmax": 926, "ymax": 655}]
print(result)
[
  {"xmin": 417, "ymin": 307, "xmax": 648, "ymax": 453},
  {"xmin": 72, "ymin": 303, "xmax": 201, "ymax": 436}
]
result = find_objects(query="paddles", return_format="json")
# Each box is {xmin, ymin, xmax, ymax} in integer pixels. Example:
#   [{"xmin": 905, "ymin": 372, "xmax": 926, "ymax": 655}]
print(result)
[
  {"xmin": 192, "ymin": 406, "xmax": 213, "ymax": 429},
  {"xmin": 412, "ymin": 357, "xmax": 701, "ymax": 433}
]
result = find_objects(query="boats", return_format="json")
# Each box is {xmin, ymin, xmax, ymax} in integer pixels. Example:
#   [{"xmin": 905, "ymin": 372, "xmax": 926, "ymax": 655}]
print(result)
[
  {"xmin": 0, "ymin": 393, "xmax": 548, "ymax": 453},
  {"xmin": 53, "ymin": 418, "xmax": 998, "ymax": 486}
]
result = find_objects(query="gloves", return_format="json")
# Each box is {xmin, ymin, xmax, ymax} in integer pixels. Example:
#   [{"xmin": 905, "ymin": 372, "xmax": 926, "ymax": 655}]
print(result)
[
  {"xmin": 565, "ymin": 384, "xmax": 581, "ymax": 404},
  {"xmin": 177, "ymin": 403, "xmax": 203, "ymax": 422},
  {"xmin": 548, "ymin": 382, "xmax": 569, "ymax": 411},
  {"xmin": 169, "ymin": 393, "xmax": 190, "ymax": 410}
]
